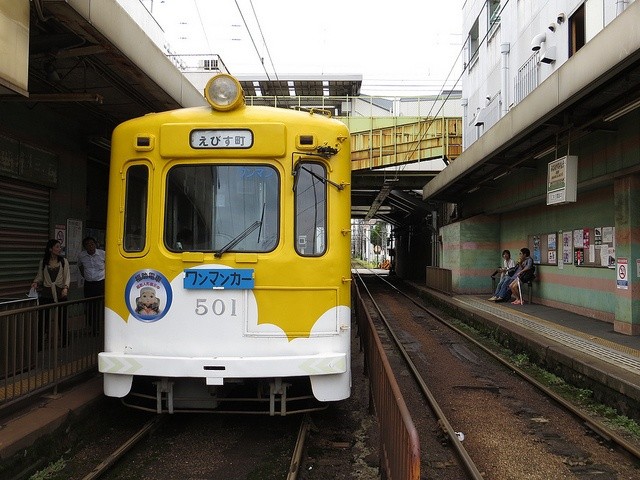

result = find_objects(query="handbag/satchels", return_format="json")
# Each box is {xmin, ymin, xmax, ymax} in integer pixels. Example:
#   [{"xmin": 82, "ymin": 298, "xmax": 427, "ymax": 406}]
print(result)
[
  {"xmin": 520, "ymin": 258, "xmax": 536, "ymax": 283},
  {"xmin": 507, "ymin": 262, "xmax": 520, "ymax": 276}
]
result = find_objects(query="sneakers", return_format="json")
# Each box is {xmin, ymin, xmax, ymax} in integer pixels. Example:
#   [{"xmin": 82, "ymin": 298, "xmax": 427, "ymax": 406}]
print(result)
[
  {"xmin": 514, "ymin": 300, "xmax": 524, "ymax": 304},
  {"xmin": 488, "ymin": 296, "xmax": 497, "ymax": 301},
  {"xmin": 511, "ymin": 300, "xmax": 517, "ymax": 305}
]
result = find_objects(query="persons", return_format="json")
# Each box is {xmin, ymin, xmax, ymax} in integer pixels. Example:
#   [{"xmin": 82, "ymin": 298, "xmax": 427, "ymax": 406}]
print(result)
[
  {"xmin": 134, "ymin": 287, "xmax": 161, "ymax": 316},
  {"xmin": 509, "ymin": 248, "xmax": 534, "ymax": 305},
  {"xmin": 77, "ymin": 237, "xmax": 105, "ymax": 337},
  {"xmin": 487, "ymin": 249, "xmax": 515, "ymax": 301},
  {"xmin": 31, "ymin": 239, "xmax": 71, "ymax": 353}
]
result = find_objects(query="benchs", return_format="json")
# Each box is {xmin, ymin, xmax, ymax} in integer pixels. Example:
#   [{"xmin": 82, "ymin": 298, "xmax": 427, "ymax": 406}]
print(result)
[{"xmin": 490, "ymin": 265, "xmax": 540, "ymax": 304}]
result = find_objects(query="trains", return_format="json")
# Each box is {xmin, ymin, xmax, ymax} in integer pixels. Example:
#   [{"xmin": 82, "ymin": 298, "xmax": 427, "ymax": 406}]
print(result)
[{"xmin": 97, "ymin": 74, "xmax": 352, "ymax": 418}]
